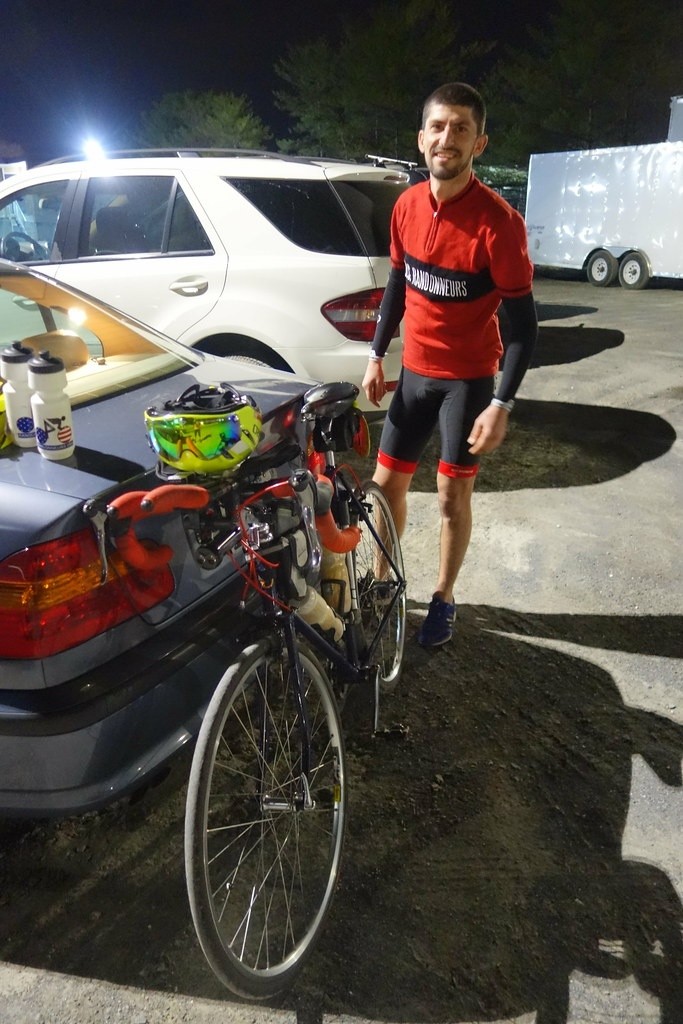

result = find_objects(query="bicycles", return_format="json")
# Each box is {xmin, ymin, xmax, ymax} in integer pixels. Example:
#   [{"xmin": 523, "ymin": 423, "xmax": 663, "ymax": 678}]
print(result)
[{"xmin": 80, "ymin": 380, "xmax": 410, "ymax": 1000}]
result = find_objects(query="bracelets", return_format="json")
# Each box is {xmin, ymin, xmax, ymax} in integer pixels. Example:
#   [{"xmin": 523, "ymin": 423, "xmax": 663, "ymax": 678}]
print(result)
[
  {"xmin": 491, "ymin": 398, "xmax": 515, "ymax": 411},
  {"xmin": 368, "ymin": 355, "xmax": 383, "ymax": 363}
]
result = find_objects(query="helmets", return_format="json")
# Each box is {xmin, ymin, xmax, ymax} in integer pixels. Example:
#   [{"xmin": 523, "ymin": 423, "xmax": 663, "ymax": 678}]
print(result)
[{"xmin": 144, "ymin": 381, "xmax": 263, "ymax": 476}]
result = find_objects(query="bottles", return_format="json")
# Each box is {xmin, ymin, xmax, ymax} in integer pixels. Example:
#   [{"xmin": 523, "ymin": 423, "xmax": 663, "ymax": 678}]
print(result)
[
  {"xmin": 287, "ymin": 586, "xmax": 344, "ymax": 645},
  {"xmin": 321, "ymin": 542, "xmax": 351, "ymax": 613},
  {"xmin": 0, "ymin": 340, "xmax": 76, "ymax": 462}
]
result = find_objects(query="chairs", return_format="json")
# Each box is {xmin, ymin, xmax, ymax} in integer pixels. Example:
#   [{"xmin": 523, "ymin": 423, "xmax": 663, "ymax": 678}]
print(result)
[
  {"xmin": 94, "ymin": 206, "xmax": 138, "ymax": 252},
  {"xmin": 18, "ymin": 330, "xmax": 88, "ymax": 369}
]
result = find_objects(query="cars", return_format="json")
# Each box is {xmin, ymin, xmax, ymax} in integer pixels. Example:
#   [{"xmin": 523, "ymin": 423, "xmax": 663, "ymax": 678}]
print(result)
[{"xmin": 0, "ymin": 254, "xmax": 325, "ymax": 822}]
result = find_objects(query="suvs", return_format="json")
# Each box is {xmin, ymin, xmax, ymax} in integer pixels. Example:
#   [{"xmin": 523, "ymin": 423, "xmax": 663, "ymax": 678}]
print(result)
[{"xmin": 0, "ymin": 146, "xmax": 413, "ymax": 443}]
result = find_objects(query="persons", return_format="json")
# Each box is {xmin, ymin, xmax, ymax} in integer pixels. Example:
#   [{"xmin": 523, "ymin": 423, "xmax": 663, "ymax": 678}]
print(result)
[{"xmin": 362, "ymin": 83, "xmax": 536, "ymax": 645}]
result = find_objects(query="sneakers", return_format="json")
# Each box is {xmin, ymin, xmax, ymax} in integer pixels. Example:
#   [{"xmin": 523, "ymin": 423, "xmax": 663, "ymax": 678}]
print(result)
[
  {"xmin": 418, "ymin": 592, "xmax": 456, "ymax": 646},
  {"xmin": 357, "ymin": 572, "xmax": 400, "ymax": 612}
]
